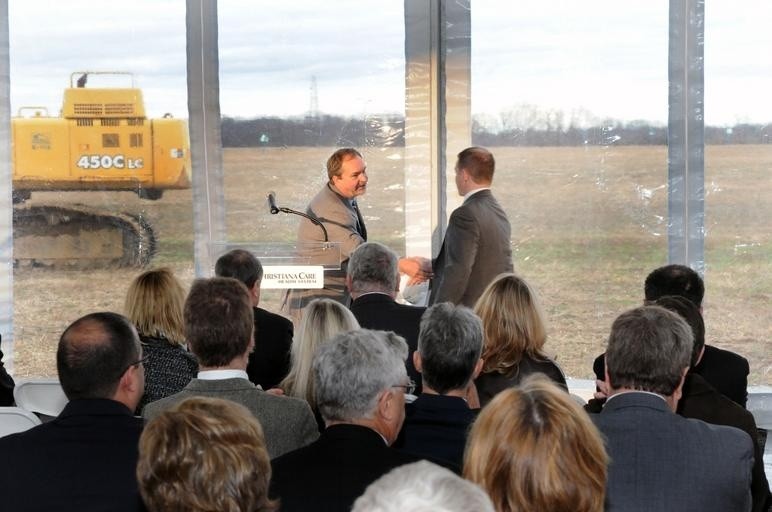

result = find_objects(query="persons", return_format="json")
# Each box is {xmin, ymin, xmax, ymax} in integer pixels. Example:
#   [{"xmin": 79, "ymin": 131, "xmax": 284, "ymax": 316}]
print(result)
[
  {"xmin": 407, "ymin": 145, "xmax": 512, "ymax": 309},
  {"xmin": 288, "ymin": 147, "xmax": 437, "ymax": 316},
  {"xmin": 120, "ymin": 237, "xmax": 573, "ymax": 511},
  {"xmin": 0, "ymin": 332, "xmax": 56, "ymax": 425},
  {"xmin": 574, "ymin": 306, "xmax": 757, "ymax": 511},
  {"xmin": 0, "ymin": 308, "xmax": 147, "ymax": 512},
  {"xmin": 586, "ymin": 297, "xmax": 771, "ymax": 512},
  {"xmin": 593, "ymin": 263, "xmax": 749, "ymax": 406}
]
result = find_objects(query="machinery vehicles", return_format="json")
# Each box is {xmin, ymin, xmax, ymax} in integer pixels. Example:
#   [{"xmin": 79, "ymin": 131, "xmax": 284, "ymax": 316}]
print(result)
[{"xmin": 12, "ymin": 70, "xmax": 192, "ymax": 269}]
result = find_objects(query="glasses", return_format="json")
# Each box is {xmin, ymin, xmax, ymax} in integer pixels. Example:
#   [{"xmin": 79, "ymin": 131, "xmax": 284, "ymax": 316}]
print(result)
[
  {"xmin": 392, "ymin": 380, "xmax": 417, "ymax": 396},
  {"xmin": 118, "ymin": 350, "xmax": 154, "ymax": 381}
]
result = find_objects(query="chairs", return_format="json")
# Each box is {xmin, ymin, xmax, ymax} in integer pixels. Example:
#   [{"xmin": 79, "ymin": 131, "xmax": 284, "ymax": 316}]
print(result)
[
  {"xmin": 404, "ymin": 389, "xmax": 590, "ymax": 410},
  {"xmin": 13, "ymin": 378, "xmax": 70, "ymax": 417},
  {"xmin": 1, "ymin": 406, "xmax": 42, "ymax": 439}
]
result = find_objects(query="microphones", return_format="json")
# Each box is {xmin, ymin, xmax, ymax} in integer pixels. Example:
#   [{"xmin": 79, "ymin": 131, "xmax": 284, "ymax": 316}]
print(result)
[{"xmin": 265, "ymin": 190, "xmax": 277, "ymax": 214}]
